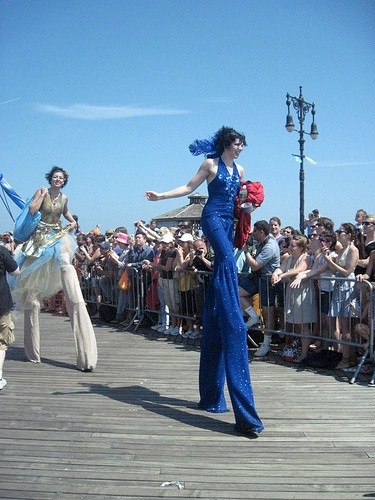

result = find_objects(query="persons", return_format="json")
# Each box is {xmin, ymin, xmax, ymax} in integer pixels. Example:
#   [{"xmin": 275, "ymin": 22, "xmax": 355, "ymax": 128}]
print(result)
[
  {"xmin": 21, "ymin": 166, "xmax": 99, "ymax": 372},
  {"xmin": 145, "ymin": 126, "xmax": 264, "ymax": 436},
  {"xmin": 0, "ymin": 208, "xmax": 375, "ymax": 389}
]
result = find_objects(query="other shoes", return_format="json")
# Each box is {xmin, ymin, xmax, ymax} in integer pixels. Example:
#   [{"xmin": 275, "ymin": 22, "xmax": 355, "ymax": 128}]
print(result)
[
  {"xmin": 293, "ymin": 356, "xmax": 308, "ymax": 363},
  {"xmin": 255, "ymin": 344, "xmax": 271, "ymax": 357},
  {"xmin": 90, "ymin": 313, "xmax": 132, "ymax": 326},
  {"xmin": 335, "ymin": 358, "xmax": 352, "ymax": 369},
  {"xmin": 313, "ymin": 346, "xmax": 327, "ymax": 352},
  {"xmin": 0, "ymin": 378, "xmax": 6, "ymax": 389},
  {"xmin": 245, "ymin": 316, "xmax": 259, "ymax": 328},
  {"xmin": 42, "ymin": 307, "xmax": 66, "ymax": 314}
]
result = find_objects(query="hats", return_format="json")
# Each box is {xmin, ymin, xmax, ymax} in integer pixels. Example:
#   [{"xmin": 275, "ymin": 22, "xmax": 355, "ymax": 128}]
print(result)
[
  {"xmin": 178, "ymin": 233, "xmax": 193, "ymax": 243},
  {"xmin": 156, "ymin": 234, "xmax": 172, "ymax": 243},
  {"xmin": 105, "ymin": 230, "xmax": 114, "ymax": 233},
  {"xmin": 99, "ymin": 241, "xmax": 110, "ymax": 249},
  {"xmin": 116, "ymin": 232, "xmax": 129, "ymax": 244}
]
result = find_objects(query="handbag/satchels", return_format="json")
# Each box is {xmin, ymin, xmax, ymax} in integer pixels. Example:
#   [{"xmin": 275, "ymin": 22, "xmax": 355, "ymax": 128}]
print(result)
[{"xmin": 117, "ymin": 270, "xmax": 131, "ymax": 290}]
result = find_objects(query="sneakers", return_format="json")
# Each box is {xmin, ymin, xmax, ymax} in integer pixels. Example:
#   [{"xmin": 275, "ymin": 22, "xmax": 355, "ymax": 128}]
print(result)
[{"xmin": 151, "ymin": 324, "xmax": 200, "ymax": 339}]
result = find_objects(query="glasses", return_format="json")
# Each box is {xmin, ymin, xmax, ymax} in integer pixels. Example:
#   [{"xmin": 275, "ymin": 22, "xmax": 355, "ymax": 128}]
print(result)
[
  {"xmin": 362, "ymin": 222, "xmax": 374, "ymax": 226},
  {"xmin": 310, "ymin": 226, "xmax": 317, "ymax": 228},
  {"xmin": 336, "ymin": 230, "xmax": 347, "ymax": 235}
]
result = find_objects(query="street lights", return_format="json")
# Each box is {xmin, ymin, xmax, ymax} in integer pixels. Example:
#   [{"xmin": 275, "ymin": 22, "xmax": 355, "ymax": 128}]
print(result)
[{"xmin": 285, "ymin": 85, "xmax": 318, "ymax": 235}]
